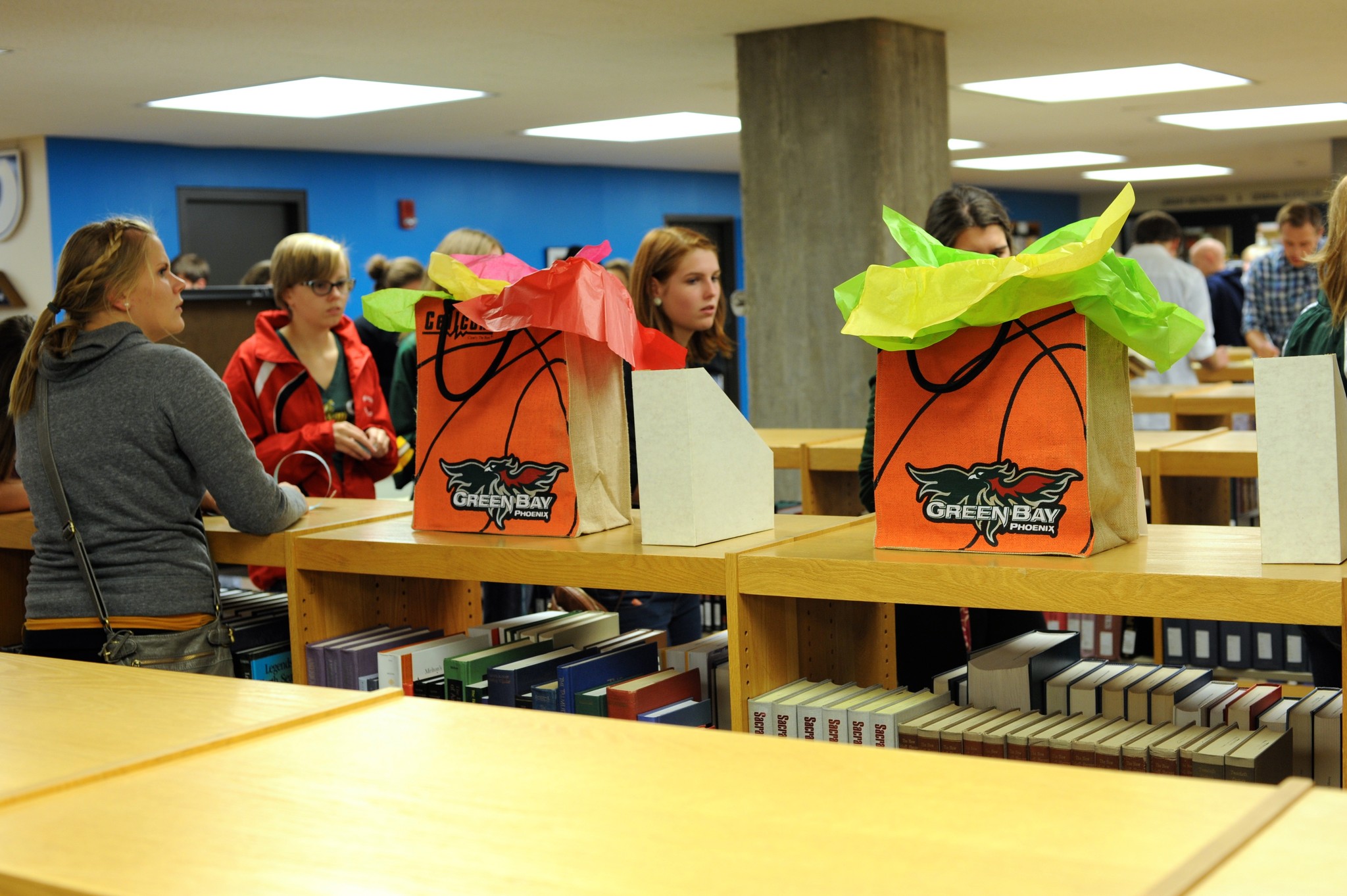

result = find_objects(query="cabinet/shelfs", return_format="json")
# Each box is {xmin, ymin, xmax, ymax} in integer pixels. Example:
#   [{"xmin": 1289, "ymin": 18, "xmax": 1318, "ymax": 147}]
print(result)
[{"xmin": 1, "ymin": 351, "xmax": 1347, "ymax": 895}]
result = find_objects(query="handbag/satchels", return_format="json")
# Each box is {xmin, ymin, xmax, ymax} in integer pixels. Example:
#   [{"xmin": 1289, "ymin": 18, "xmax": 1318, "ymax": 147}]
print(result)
[
  {"xmin": 412, "ymin": 296, "xmax": 633, "ymax": 538},
  {"xmin": 876, "ymin": 306, "xmax": 1138, "ymax": 555},
  {"xmin": 97, "ymin": 606, "xmax": 235, "ymax": 679}
]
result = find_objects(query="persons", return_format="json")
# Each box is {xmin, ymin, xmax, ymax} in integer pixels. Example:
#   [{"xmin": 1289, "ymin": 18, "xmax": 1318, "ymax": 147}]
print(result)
[
  {"xmin": 1282, "ymin": 175, "xmax": 1347, "ymax": 395},
  {"xmin": 1126, "ymin": 210, "xmax": 1226, "ymax": 430},
  {"xmin": 241, "ymin": 262, "xmax": 272, "ymax": 284},
  {"xmin": 9, "ymin": 217, "xmax": 308, "ymax": 662},
  {"xmin": 0, "ymin": 316, "xmax": 36, "ymax": 480},
  {"xmin": 859, "ymin": 185, "xmax": 1047, "ymax": 691},
  {"xmin": 224, "ymin": 233, "xmax": 399, "ymax": 593},
  {"xmin": 1191, "ymin": 200, "xmax": 1325, "ymax": 385},
  {"xmin": 171, "ymin": 254, "xmax": 210, "ymax": 288},
  {"xmin": 392, "ymin": 228, "xmax": 532, "ymax": 623},
  {"xmin": 604, "ymin": 227, "xmax": 734, "ymax": 645},
  {"xmin": 354, "ymin": 254, "xmax": 423, "ymax": 406}
]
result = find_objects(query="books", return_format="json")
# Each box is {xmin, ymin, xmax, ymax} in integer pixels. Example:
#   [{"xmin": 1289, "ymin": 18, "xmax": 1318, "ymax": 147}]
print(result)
[
  {"xmin": 219, "ymin": 586, "xmax": 293, "ymax": 683},
  {"xmin": 746, "ymin": 470, "xmax": 1343, "ymax": 788},
  {"xmin": 307, "ymin": 594, "xmax": 731, "ymax": 730}
]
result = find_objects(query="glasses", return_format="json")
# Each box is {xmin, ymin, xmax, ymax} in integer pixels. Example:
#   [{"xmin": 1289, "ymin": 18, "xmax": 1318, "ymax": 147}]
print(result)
[{"xmin": 288, "ymin": 278, "xmax": 355, "ymax": 296}]
